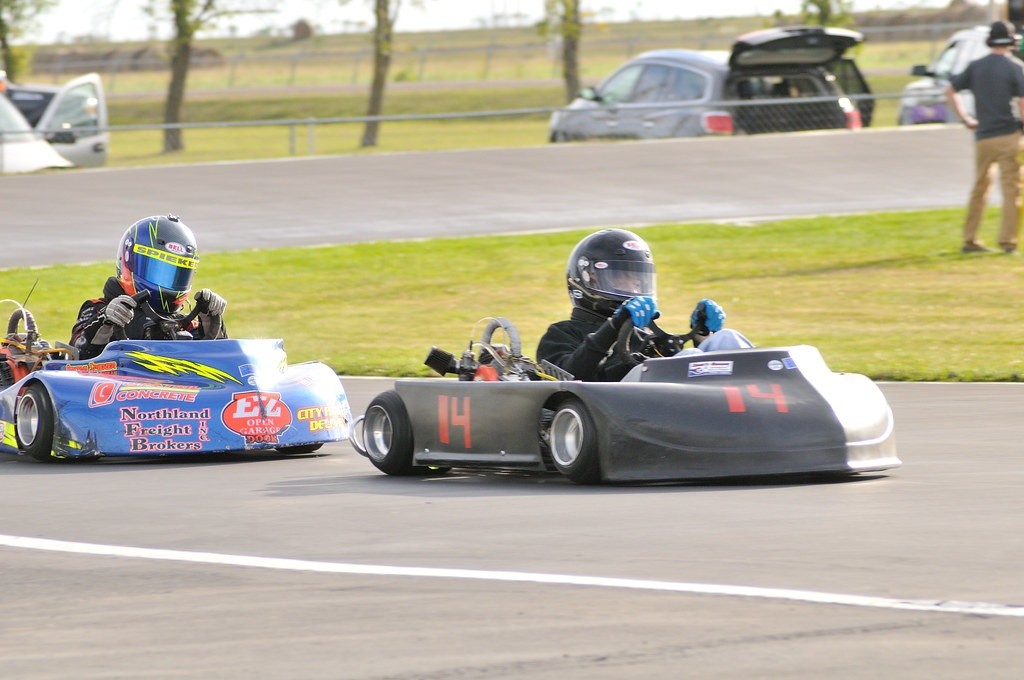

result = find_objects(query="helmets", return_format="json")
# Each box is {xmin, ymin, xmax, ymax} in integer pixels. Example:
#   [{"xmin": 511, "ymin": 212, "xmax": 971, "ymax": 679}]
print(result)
[
  {"xmin": 113, "ymin": 213, "xmax": 200, "ymax": 310},
  {"xmin": 566, "ymin": 229, "xmax": 656, "ymax": 315}
]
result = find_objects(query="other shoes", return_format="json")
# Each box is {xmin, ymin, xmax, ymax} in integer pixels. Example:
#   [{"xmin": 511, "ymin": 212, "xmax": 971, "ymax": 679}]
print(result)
[
  {"xmin": 962, "ymin": 241, "xmax": 992, "ymax": 254},
  {"xmin": 1000, "ymin": 243, "xmax": 1018, "ymax": 251}
]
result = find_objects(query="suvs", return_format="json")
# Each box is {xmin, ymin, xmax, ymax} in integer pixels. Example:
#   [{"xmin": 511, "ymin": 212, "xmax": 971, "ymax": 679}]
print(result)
[{"xmin": 547, "ymin": 23, "xmax": 878, "ymax": 143}]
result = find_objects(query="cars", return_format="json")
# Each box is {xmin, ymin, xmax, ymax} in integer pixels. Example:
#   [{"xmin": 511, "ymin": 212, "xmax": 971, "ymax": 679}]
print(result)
[
  {"xmin": 898, "ymin": 26, "xmax": 1024, "ymax": 126},
  {"xmin": 0, "ymin": 91, "xmax": 76, "ymax": 171},
  {"xmin": 4, "ymin": 71, "xmax": 109, "ymax": 168}
]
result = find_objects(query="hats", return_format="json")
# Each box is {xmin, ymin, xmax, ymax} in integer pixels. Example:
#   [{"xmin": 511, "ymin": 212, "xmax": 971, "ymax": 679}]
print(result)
[{"xmin": 986, "ymin": 21, "xmax": 1017, "ymax": 47}]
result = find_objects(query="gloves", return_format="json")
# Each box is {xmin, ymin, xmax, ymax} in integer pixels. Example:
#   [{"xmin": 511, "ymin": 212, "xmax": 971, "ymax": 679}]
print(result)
[
  {"xmin": 96, "ymin": 294, "xmax": 137, "ymax": 327},
  {"xmin": 613, "ymin": 295, "xmax": 658, "ymax": 328},
  {"xmin": 690, "ymin": 298, "xmax": 726, "ymax": 333},
  {"xmin": 200, "ymin": 288, "xmax": 228, "ymax": 317}
]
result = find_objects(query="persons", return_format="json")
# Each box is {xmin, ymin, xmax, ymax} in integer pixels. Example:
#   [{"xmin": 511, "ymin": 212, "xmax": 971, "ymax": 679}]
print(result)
[
  {"xmin": 69, "ymin": 214, "xmax": 227, "ymax": 361},
  {"xmin": 535, "ymin": 228, "xmax": 727, "ymax": 383},
  {"xmin": 944, "ymin": 20, "xmax": 1024, "ymax": 255}
]
